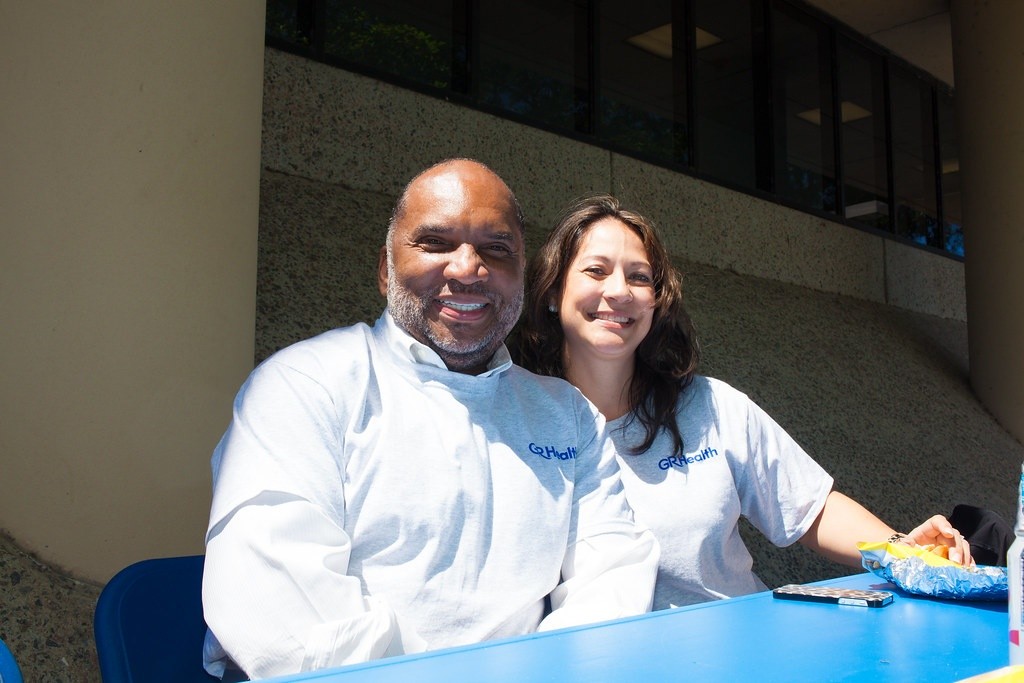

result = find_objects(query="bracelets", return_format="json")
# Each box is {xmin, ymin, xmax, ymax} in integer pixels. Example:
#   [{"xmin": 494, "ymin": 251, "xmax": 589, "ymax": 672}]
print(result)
[{"xmin": 888, "ymin": 533, "xmax": 907, "ymax": 544}]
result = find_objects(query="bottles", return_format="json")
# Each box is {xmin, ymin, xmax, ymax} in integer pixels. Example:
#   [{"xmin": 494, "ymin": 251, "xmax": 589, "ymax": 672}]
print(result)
[{"xmin": 1007, "ymin": 462, "xmax": 1024, "ymax": 666}]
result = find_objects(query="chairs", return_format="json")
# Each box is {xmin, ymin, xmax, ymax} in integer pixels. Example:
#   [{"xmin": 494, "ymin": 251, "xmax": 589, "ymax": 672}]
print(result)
[{"xmin": 93, "ymin": 554, "xmax": 221, "ymax": 683}]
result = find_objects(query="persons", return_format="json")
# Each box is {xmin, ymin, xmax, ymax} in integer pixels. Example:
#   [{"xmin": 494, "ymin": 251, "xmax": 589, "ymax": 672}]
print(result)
[
  {"xmin": 510, "ymin": 196, "xmax": 977, "ymax": 612},
  {"xmin": 202, "ymin": 159, "xmax": 662, "ymax": 681}
]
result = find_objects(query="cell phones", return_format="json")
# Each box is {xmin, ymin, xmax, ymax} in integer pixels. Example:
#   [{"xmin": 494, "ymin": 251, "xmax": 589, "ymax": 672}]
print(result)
[{"xmin": 772, "ymin": 584, "xmax": 894, "ymax": 608}]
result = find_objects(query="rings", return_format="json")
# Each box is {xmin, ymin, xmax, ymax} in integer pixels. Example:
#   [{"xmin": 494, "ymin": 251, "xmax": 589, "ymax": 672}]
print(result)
[{"xmin": 961, "ymin": 535, "xmax": 964, "ymax": 539}]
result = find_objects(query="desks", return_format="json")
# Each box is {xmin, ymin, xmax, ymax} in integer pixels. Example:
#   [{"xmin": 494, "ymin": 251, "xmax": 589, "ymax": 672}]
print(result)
[{"xmin": 234, "ymin": 564, "xmax": 1008, "ymax": 683}]
